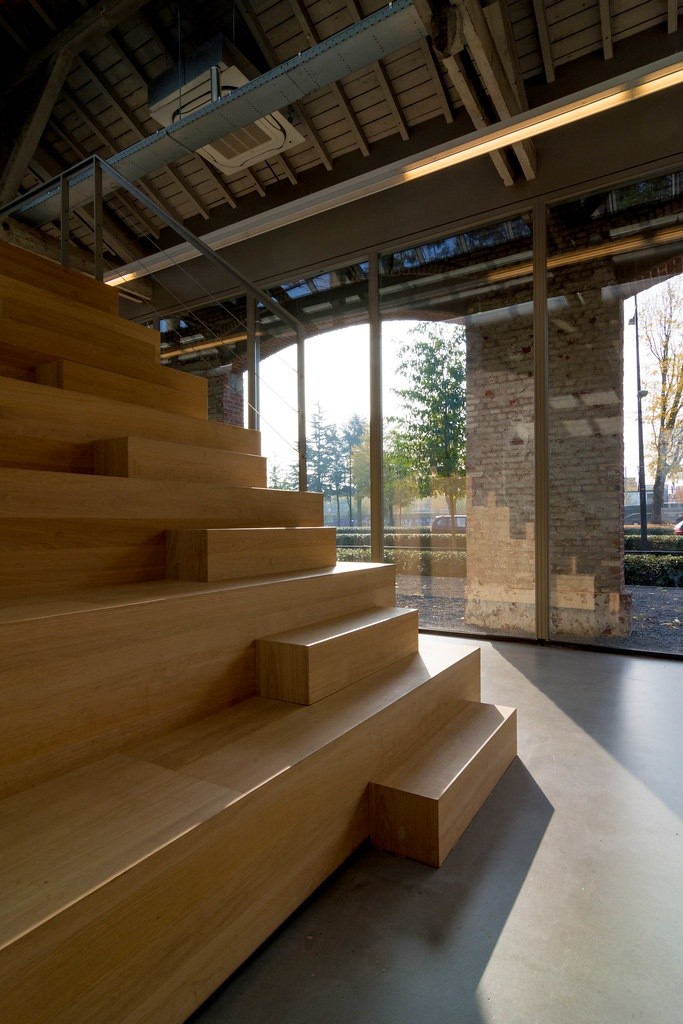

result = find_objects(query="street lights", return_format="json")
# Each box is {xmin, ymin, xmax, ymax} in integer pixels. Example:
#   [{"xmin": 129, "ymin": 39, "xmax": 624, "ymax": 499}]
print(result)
[{"xmin": 629, "ymin": 292, "xmax": 647, "ymax": 554}]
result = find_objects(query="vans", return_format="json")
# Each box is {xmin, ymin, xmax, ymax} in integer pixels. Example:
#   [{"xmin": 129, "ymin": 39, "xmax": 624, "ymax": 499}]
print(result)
[{"xmin": 431, "ymin": 514, "xmax": 466, "ymax": 535}]
